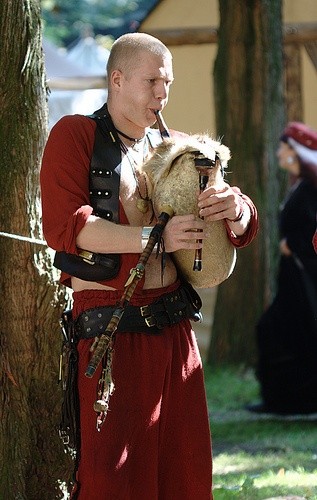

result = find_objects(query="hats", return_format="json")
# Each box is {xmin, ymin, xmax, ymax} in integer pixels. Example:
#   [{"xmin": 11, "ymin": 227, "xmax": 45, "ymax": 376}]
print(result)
[{"xmin": 279, "ymin": 121, "xmax": 316, "ymax": 176}]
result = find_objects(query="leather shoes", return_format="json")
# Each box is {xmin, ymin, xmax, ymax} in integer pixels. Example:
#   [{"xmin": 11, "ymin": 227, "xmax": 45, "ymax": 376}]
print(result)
[{"xmin": 61, "ymin": 280, "xmax": 206, "ymax": 340}]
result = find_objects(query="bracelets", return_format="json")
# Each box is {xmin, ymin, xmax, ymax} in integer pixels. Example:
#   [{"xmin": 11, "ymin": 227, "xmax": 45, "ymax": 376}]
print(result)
[
  {"xmin": 226, "ymin": 197, "xmax": 244, "ymax": 222},
  {"xmin": 141, "ymin": 226, "xmax": 162, "ymax": 255}
]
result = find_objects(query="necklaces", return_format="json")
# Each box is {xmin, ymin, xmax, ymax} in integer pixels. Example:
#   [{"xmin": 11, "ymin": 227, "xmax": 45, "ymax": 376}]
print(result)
[
  {"xmin": 116, "ymin": 134, "xmax": 155, "ymax": 225},
  {"xmin": 115, "ymin": 128, "xmax": 146, "ymax": 152}
]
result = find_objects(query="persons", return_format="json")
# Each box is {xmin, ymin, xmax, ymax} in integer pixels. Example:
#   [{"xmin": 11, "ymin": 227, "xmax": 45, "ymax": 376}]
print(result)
[
  {"xmin": 40, "ymin": 32, "xmax": 259, "ymax": 500},
  {"xmin": 242, "ymin": 121, "xmax": 317, "ymax": 414}
]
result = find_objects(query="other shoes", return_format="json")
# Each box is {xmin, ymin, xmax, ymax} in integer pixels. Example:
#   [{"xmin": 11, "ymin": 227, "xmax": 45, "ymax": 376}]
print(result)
[{"xmin": 255, "ymin": 403, "xmax": 316, "ymax": 416}]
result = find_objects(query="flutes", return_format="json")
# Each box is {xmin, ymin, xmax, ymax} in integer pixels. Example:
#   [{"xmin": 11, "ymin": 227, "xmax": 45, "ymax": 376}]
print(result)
[
  {"xmin": 153, "ymin": 108, "xmax": 171, "ymax": 140},
  {"xmin": 85, "ymin": 211, "xmax": 171, "ymax": 379},
  {"xmin": 193, "ymin": 158, "xmax": 216, "ymax": 271}
]
